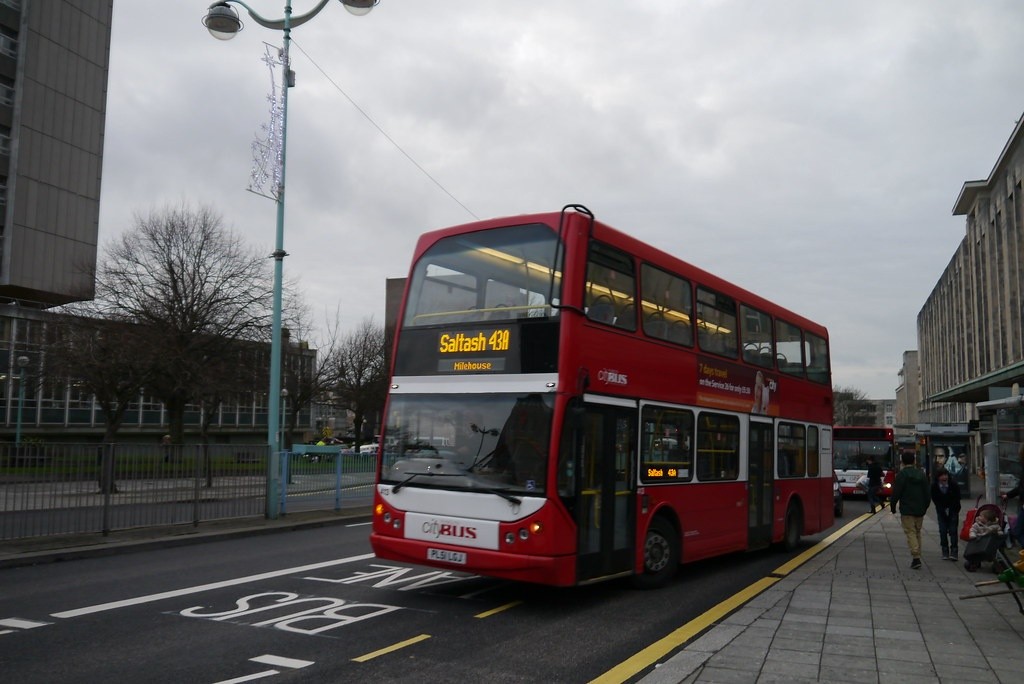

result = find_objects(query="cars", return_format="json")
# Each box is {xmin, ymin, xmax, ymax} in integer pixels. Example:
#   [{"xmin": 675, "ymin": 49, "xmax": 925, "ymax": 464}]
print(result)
[
  {"xmin": 301, "ymin": 430, "xmax": 457, "ymax": 473},
  {"xmin": 828, "ymin": 471, "xmax": 846, "ymax": 516}
]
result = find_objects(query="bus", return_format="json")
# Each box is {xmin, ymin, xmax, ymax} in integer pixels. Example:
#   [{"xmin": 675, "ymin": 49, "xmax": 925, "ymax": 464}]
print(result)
[
  {"xmin": 369, "ymin": 201, "xmax": 839, "ymax": 596},
  {"xmin": 828, "ymin": 424, "xmax": 897, "ymax": 495}
]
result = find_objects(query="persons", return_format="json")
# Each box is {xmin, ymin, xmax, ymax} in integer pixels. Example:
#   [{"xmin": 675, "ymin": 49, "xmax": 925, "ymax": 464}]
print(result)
[
  {"xmin": 751, "ymin": 371, "xmax": 768, "ymax": 415},
  {"xmin": 856, "ymin": 456, "xmax": 885, "ymax": 514},
  {"xmin": 932, "ymin": 446, "xmax": 950, "ymax": 476},
  {"xmin": 1002, "ymin": 443, "xmax": 1024, "ymax": 550},
  {"xmin": 456, "ymin": 409, "xmax": 518, "ymax": 486},
  {"xmin": 932, "ymin": 468, "xmax": 959, "ymax": 561},
  {"xmin": 890, "ymin": 452, "xmax": 931, "ymax": 569},
  {"xmin": 969, "ymin": 510, "xmax": 1003, "ymax": 538},
  {"xmin": 951, "ymin": 446, "xmax": 967, "ymax": 477}
]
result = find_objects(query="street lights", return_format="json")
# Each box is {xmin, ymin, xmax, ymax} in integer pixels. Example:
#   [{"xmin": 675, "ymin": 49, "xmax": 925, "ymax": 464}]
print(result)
[
  {"xmin": 278, "ymin": 388, "xmax": 289, "ymax": 450},
  {"xmin": 14, "ymin": 356, "xmax": 30, "ymax": 446},
  {"xmin": 205, "ymin": 0, "xmax": 385, "ymax": 525}
]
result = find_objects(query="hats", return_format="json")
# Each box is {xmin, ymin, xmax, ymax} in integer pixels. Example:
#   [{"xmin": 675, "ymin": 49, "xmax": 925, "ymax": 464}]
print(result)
[{"xmin": 935, "ymin": 465, "xmax": 948, "ymax": 476}]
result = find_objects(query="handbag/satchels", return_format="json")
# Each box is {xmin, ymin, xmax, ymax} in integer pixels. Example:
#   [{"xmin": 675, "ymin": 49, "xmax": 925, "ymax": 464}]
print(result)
[{"xmin": 963, "ymin": 533, "xmax": 1000, "ymax": 562}]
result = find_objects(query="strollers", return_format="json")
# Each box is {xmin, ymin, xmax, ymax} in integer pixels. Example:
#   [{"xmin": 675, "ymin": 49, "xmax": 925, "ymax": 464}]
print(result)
[{"xmin": 963, "ymin": 492, "xmax": 1008, "ymax": 575}]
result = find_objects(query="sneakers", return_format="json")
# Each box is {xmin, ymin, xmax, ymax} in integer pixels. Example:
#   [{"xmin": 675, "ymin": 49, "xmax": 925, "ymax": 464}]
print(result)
[
  {"xmin": 910, "ymin": 557, "xmax": 922, "ymax": 568},
  {"xmin": 942, "ymin": 546, "xmax": 958, "ymax": 561}
]
